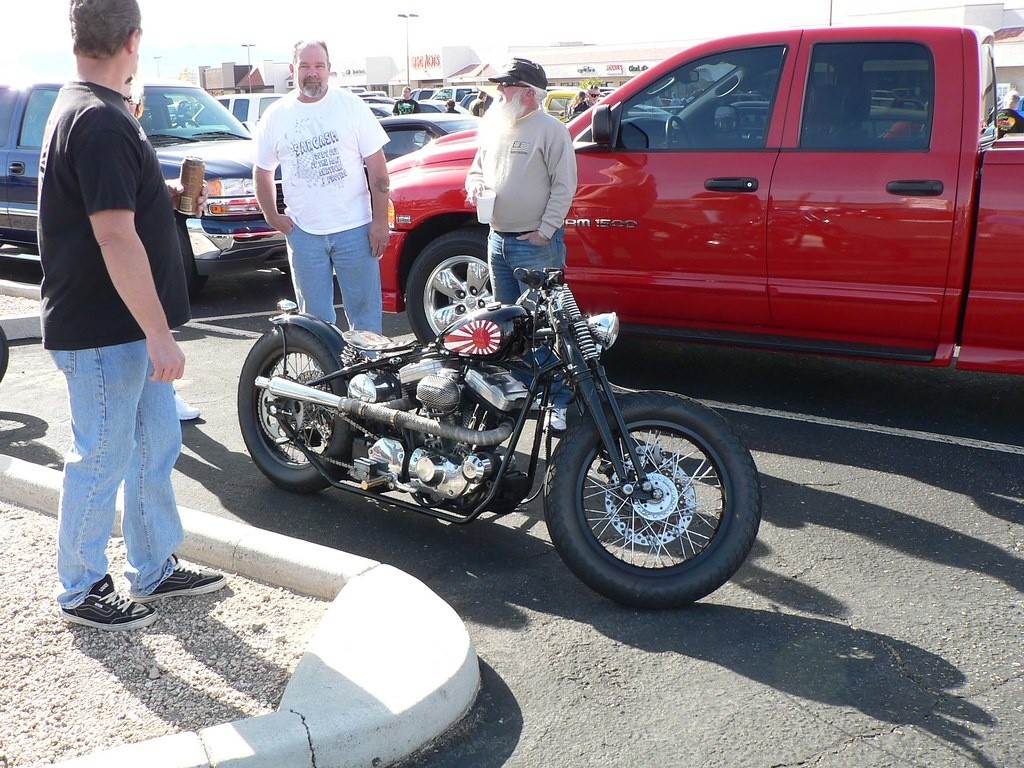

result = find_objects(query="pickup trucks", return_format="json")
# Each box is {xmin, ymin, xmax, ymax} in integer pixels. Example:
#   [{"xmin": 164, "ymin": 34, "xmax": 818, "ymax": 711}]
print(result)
[
  {"xmin": 376, "ymin": 26, "xmax": 1023, "ymax": 380},
  {"xmin": 0, "ymin": 81, "xmax": 292, "ymax": 304}
]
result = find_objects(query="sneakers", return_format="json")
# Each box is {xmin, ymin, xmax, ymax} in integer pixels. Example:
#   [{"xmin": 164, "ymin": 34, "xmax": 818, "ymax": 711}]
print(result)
[
  {"xmin": 60, "ymin": 574, "xmax": 158, "ymax": 632},
  {"xmin": 128, "ymin": 553, "xmax": 226, "ymax": 603},
  {"xmin": 173, "ymin": 390, "xmax": 201, "ymax": 420}
]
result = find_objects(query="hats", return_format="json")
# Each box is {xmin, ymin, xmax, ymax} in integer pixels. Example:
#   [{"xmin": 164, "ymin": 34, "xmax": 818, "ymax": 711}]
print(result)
[{"xmin": 488, "ymin": 57, "xmax": 548, "ymax": 93}]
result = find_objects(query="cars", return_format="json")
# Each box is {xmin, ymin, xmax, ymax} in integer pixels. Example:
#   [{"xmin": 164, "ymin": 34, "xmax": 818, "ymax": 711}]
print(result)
[
  {"xmin": 870, "ymin": 85, "xmax": 927, "ymax": 141},
  {"xmin": 163, "ymin": 84, "xmax": 769, "ymax": 166}
]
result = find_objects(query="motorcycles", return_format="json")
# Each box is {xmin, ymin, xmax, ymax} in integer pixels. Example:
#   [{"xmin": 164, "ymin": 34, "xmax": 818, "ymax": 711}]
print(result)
[{"xmin": 235, "ymin": 265, "xmax": 761, "ymax": 613}]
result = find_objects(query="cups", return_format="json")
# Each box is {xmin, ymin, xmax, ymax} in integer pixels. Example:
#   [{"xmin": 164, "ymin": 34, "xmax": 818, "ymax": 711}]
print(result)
[{"xmin": 475, "ymin": 190, "xmax": 496, "ymax": 224}]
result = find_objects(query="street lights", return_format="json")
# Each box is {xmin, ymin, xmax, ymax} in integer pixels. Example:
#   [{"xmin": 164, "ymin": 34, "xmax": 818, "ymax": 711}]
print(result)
[
  {"xmin": 397, "ymin": 13, "xmax": 419, "ymax": 87},
  {"xmin": 241, "ymin": 44, "xmax": 257, "ymax": 94},
  {"xmin": 153, "ymin": 57, "xmax": 162, "ymax": 80}
]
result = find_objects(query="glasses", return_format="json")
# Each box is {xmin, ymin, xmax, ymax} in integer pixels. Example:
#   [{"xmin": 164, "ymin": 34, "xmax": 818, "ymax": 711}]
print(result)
[
  {"xmin": 123, "ymin": 95, "xmax": 142, "ymax": 105},
  {"xmin": 588, "ymin": 93, "xmax": 601, "ymax": 98},
  {"xmin": 499, "ymin": 80, "xmax": 530, "ymax": 87}
]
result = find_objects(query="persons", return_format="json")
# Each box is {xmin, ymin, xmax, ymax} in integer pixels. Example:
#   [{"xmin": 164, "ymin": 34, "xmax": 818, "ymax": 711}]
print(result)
[
  {"xmin": 37, "ymin": 0, "xmax": 227, "ymax": 632},
  {"xmin": 670, "ymin": 92, "xmax": 681, "ymax": 106},
  {"xmin": 469, "ymin": 91, "xmax": 487, "ymax": 117},
  {"xmin": 444, "ymin": 99, "xmax": 460, "ymax": 113},
  {"xmin": 998, "ymin": 82, "xmax": 1024, "ymax": 134},
  {"xmin": 465, "ymin": 57, "xmax": 577, "ymax": 432},
  {"xmin": 392, "ymin": 86, "xmax": 420, "ymax": 116},
  {"xmin": 247, "ymin": 40, "xmax": 390, "ymax": 374},
  {"xmin": 567, "ymin": 85, "xmax": 600, "ymax": 118}
]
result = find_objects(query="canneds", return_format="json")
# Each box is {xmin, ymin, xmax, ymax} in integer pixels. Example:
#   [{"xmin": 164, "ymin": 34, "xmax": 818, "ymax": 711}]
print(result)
[{"xmin": 176, "ymin": 156, "xmax": 205, "ymax": 214}]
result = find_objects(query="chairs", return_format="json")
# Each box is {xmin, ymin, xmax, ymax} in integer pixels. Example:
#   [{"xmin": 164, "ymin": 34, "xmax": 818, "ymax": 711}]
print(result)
[{"xmin": 805, "ymin": 85, "xmax": 872, "ymax": 138}]
